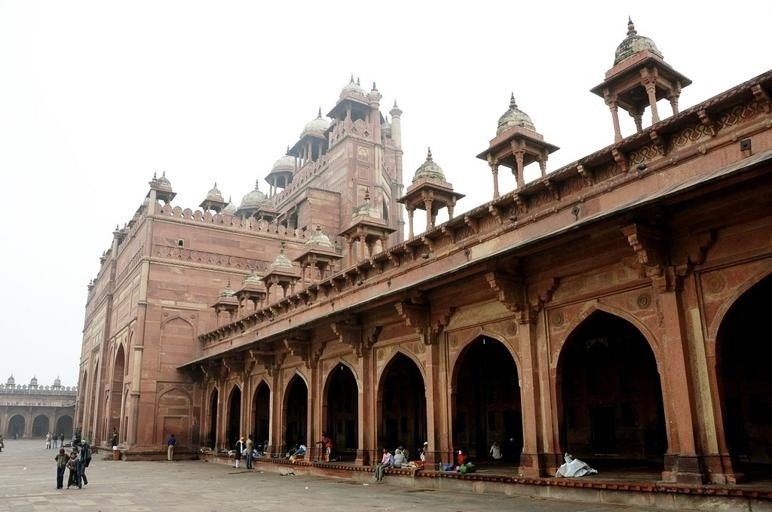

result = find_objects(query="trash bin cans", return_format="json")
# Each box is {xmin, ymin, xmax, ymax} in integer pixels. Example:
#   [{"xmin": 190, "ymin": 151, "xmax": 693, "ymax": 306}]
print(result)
[{"xmin": 113, "ymin": 450, "xmax": 120, "ymax": 460}]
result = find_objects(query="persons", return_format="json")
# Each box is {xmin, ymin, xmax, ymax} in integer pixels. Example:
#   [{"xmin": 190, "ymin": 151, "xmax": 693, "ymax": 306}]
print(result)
[
  {"xmin": 46, "ymin": 432, "xmax": 91, "ymax": 489},
  {"xmin": 112, "ymin": 427, "xmax": 120, "ymax": 448},
  {"xmin": 0, "ymin": 435, "xmax": 4, "ymax": 452},
  {"xmin": 232, "ymin": 433, "xmax": 334, "ymax": 470},
  {"xmin": 167, "ymin": 433, "xmax": 176, "ymax": 462},
  {"xmin": 372, "ymin": 441, "xmax": 428, "ymax": 485},
  {"xmin": 456, "ymin": 449, "xmax": 468, "ymax": 469},
  {"xmin": 489, "ymin": 441, "xmax": 504, "ymax": 465}
]
